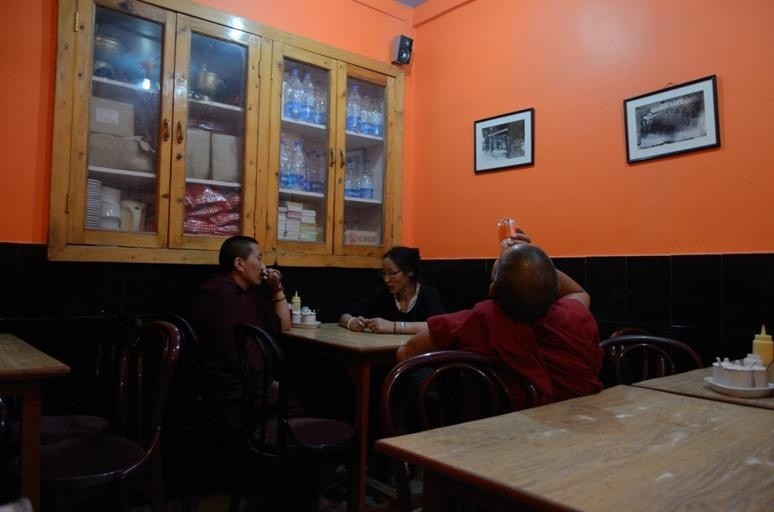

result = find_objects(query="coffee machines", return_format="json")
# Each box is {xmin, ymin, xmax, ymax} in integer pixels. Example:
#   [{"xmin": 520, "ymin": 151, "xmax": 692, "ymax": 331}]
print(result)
[{"xmin": 100, "ymin": 184, "xmax": 122, "ymax": 232}]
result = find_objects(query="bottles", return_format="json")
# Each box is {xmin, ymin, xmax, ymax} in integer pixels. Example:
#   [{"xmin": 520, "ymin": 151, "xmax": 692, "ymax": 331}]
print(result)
[
  {"xmin": 291, "ymin": 291, "xmax": 301, "ymax": 312},
  {"xmin": 284, "ymin": 66, "xmax": 385, "ymax": 141},
  {"xmin": 279, "ymin": 139, "xmax": 374, "ymax": 200},
  {"xmin": 751, "ymin": 325, "xmax": 774, "ymax": 385},
  {"xmin": 495, "ymin": 216, "xmax": 519, "ymax": 246}
]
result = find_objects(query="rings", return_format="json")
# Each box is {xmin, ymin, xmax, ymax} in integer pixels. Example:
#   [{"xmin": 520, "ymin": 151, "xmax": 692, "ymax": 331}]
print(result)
[{"xmin": 358, "ymin": 321, "xmax": 362, "ymax": 325}]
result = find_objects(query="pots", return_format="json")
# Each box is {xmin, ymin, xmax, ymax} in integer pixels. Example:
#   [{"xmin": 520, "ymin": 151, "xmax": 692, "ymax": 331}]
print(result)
[
  {"xmin": 190, "ymin": 62, "xmax": 228, "ymax": 99},
  {"xmin": 93, "ymin": 32, "xmax": 131, "ymax": 77}
]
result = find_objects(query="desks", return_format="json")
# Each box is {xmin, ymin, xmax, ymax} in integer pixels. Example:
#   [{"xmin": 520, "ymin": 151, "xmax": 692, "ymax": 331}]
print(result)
[
  {"xmin": 635, "ymin": 356, "xmax": 774, "ymax": 409},
  {"xmin": 375, "ymin": 384, "xmax": 773, "ymax": 512},
  {"xmin": 263, "ymin": 311, "xmax": 422, "ymax": 512},
  {"xmin": 0, "ymin": 331, "xmax": 71, "ymax": 511}
]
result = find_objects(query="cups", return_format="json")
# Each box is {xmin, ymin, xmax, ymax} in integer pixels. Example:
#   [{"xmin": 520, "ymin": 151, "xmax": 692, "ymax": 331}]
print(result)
[
  {"xmin": 292, "ymin": 313, "xmax": 317, "ymax": 324},
  {"xmin": 712, "ymin": 356, "xmax": 768, "ymax": 388}
]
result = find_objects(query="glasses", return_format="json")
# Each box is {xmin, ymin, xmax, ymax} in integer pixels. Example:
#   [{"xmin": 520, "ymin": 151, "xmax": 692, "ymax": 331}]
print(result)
[{"xmin": 378, "ymin": 270, "xmax": 403, "ymax": 278}]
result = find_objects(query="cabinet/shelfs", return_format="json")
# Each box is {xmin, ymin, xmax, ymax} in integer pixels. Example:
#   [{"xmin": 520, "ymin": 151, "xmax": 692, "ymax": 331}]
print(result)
[
  {"xmin": 261, "ymin": 30, "xmax": 404, "ymax": 269},
  {"xmin": 47, "ymin": 0, "xmax": 275, "ymax": 270}
]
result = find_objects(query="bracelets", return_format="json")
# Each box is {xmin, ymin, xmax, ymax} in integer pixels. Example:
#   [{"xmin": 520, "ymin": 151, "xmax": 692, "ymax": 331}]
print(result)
[
  {"xmin": 347, "ymin": 317, "xmax": 356, "ymax": 328},
  {"xmin": 400, "ymin": 321, "xmax": 407, "ymax": 333},
  {"xmin": 394, "ymin": 322, "xmax": 396, "ymax": 333},
  {"xmin": 272, "ymin": 287, "xmax": 284, "ymax": 293},
  {"xmin": 271, "ymin": 295, "xmax": 286, "ymax": 303}
]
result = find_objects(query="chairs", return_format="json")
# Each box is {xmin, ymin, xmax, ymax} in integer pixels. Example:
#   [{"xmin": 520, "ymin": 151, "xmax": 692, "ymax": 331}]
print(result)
[
  {"xmin": 597, "ymin": 335, "xmax": 702, "ymax": 385},
  {"xmin": 165, "ymin": 310, "xmax": 239, "ymax": 511},
  {"xmin": 8, "ymin": 319, "xmax": 182, "ymax": 511},
  {"xmin": 225, "ymin": 320, "xmax": 358, "ymax": 512},
  {"xmin": 5, "ymin": 306, "xmax": 145, "ymax": 454},
  {"xmin": 380, "ymin": 351, "xmax": 539, "ymax": 511}
]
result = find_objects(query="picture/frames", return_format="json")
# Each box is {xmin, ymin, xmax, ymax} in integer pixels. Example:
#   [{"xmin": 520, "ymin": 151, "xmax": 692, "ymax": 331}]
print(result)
[
  {"xmin": 473, "ymin": 107, "xmax": 534, "ymax": 174},
  {"xmin": 622, "ymin": 73, "xmax": 722, "ymax": 164}
]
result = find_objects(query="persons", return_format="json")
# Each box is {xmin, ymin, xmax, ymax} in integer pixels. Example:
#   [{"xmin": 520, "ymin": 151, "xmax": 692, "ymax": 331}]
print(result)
[
  {"xmin": 340, "ymin": 247, "xmax": 446, "ymax": 333},
  {"xmin": 191, "ymin": 235, "xmax": 356, "ymax": 422},
  {"xmin": 397, "ymin": 227, "xmax": 605, "ymax": 421}
]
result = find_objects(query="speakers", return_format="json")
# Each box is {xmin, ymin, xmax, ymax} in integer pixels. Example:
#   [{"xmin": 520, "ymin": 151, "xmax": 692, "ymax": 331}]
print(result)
[{"xmin": 392, "ymin": 34, "xmax": 413, "ymax": 65}]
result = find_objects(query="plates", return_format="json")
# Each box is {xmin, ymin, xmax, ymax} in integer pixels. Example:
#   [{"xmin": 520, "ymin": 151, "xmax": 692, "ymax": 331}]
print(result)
[
  {"xmin": 702, "ymin": 375, "xmax": 774, "ymax": 398},
  {"xmin": 293, "ymin": 322, "xmax": 321, "ymax": 329},
  {"xmin": 84, "ymin": 178, "xmax": 104, "ymax": 229}
]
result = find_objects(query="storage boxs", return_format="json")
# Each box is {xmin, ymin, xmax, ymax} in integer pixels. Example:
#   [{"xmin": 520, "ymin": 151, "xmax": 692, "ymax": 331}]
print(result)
[{"xmin": 85, "ymin": 91, "xmax": 239, "ymax": 183}]
